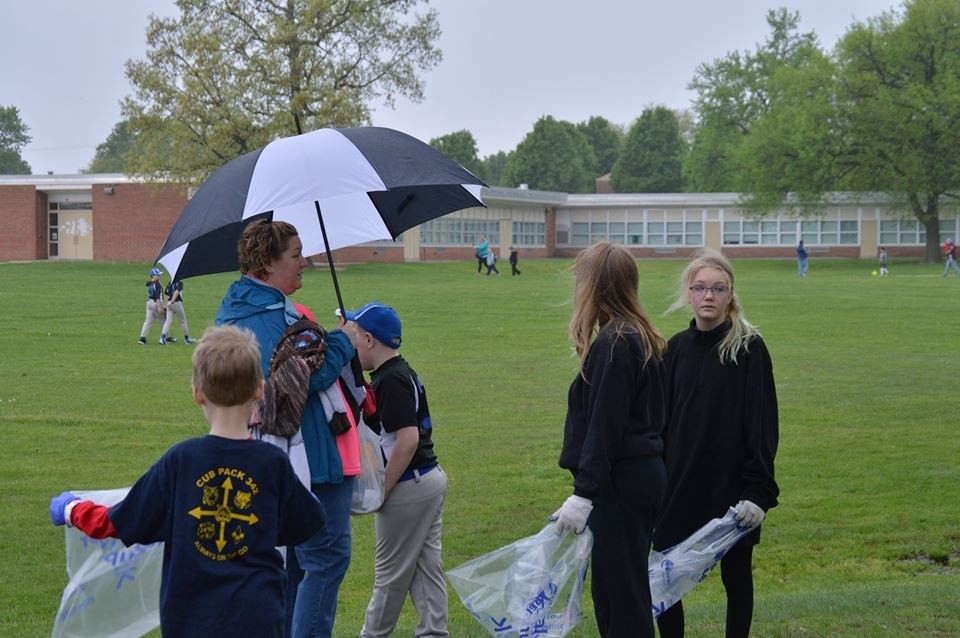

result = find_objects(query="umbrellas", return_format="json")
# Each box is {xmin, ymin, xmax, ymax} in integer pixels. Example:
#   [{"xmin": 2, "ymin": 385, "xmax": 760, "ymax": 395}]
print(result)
[{"xmin": 151, "ymin": 111, "xmax": 489, "ymax": 388}]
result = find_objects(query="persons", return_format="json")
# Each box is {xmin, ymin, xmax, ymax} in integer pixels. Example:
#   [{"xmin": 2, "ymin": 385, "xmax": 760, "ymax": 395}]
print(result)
[
  {"xmin": 137, "ymin": 268, "xmax": 196, "ymax": 345},
  {"xmin": 509, "ymin": 246, "xmax": 520, "ymax": 275},
  {"xmin": 473, "ymin": 236, "xmax": 500, "ymax": 276},
  {"xmin": 50, "ymin": 325, "xmax": 324, "ymax": 638},
  {"xmin": 213, "ymin": 217, "xmax": 374, "ymax": 638},
  {"xmin": 943, "ymin": 239, "xmax": 960, "ymax": 276},
  {"xmin": 651, "ymin": 255, "xmax": 780, "ymax": 638},
  {"xmin": 545, "ymin": 241, "xmax": 666, "ymax": 638},
  {"xmin": 879, "ymin": 247, "xmax": 889, "ymax": 274},
  {"xmin": 796, "ymin": 240, "xmax": 809, "ymax": 277},
  {"xmin": 335, "ymin": 303, "xmax": 450, "ymax": 637}
]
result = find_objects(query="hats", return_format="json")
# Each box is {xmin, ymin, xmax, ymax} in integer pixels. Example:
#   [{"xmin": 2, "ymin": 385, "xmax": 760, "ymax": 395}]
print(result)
[
  {"xmin": 149, "ymin": 267, "xmax": 163, "ymax": 277},
  {"xmin": 335, "ymin": 302, "xmax": 402, "ymax": 350}
]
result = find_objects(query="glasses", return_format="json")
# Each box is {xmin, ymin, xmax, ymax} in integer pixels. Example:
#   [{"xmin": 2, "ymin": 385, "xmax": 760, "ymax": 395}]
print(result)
[{"xmin": 690, "ymin": 284, "xmax": 729, "ymax": 296}]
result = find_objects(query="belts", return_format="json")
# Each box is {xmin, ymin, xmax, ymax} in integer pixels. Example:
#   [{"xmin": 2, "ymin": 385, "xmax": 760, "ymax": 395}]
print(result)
[{"xmin": 391, "ymin": 465, "xmax": 440, "ymax": 483}]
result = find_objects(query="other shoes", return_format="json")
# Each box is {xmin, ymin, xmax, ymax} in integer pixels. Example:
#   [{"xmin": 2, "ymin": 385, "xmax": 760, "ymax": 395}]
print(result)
[
  {"xmin": 159, "ymin": 335, "xmax": 168, "ymax": 344},
  {"xmin": 184, "ymin": 337, "xmax": 198, "ymax": 345},
  {"xmin": 166, "ymin": 336, "xmax": 177, "ymax": 342},
  {"xmin": 137, "ymin": 338, "xmax": 147, "ymax": 345}
]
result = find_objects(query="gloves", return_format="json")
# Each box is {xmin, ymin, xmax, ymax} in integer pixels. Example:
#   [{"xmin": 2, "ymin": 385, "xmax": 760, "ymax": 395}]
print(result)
[
  {"xmin": 731, "ymin": 498, "xmax": 767, "ymax": 531},
  {"xmin": 48, "ymin": 490, "xmax": 81, "ymax": 528},
  {"xmin": 549, "ymin": 495, "xmax": 594, "ymax": 537}
]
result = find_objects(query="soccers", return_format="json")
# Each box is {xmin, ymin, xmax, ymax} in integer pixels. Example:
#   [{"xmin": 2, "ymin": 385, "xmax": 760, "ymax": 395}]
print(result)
[{"xmin": 871, "ymin": 271, "xmax": 878, "ymax": 276}]
